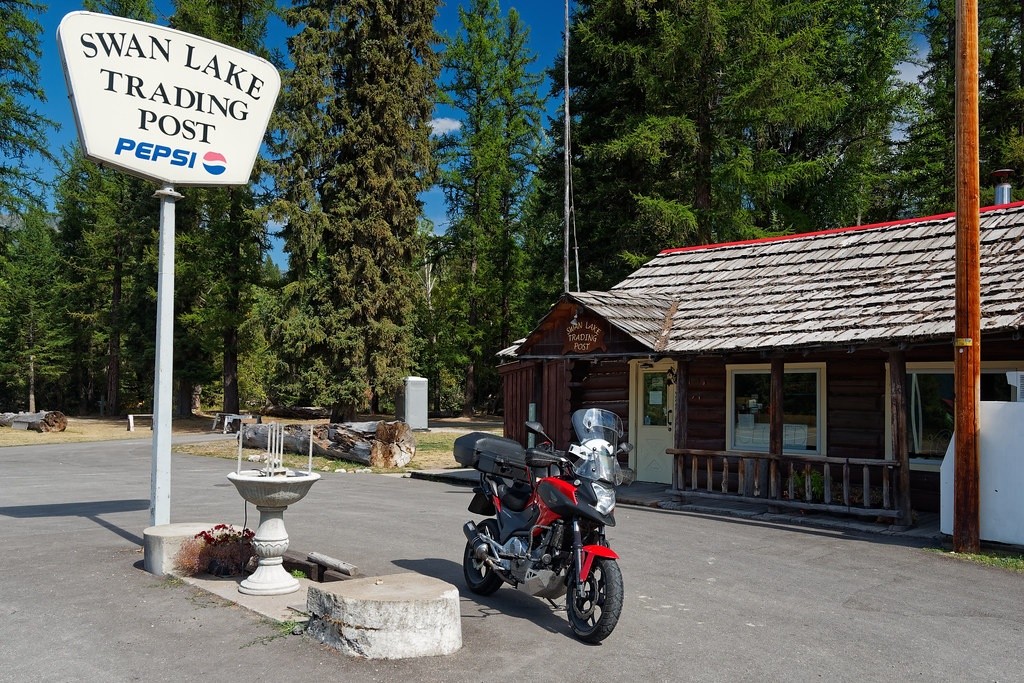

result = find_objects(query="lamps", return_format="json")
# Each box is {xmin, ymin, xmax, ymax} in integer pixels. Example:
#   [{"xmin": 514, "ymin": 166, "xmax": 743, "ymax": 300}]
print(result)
[{"xmin": 665, "ymin": 366, "xmax": 677, "ymax": 386}]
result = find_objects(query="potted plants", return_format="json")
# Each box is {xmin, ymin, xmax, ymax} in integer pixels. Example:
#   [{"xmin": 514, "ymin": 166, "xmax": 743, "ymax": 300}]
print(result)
[{"xmin": 785, "ymin": 468, "xmax": 824, "ymax": 501}]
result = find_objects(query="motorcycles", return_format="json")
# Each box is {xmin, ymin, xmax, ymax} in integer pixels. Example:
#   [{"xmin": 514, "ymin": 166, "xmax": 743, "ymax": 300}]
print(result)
[{"xmin": 453, "ymin": 407, "xmax": 636, "ymax": 644}]
